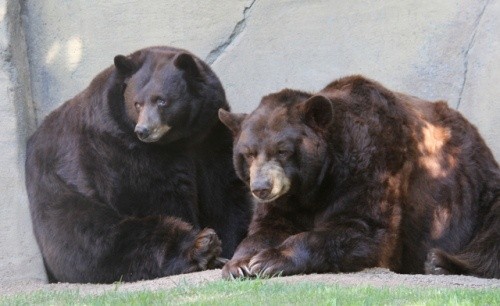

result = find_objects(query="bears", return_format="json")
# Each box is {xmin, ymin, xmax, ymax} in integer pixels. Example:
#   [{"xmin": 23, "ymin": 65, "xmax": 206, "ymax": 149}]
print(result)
[
  {"xmin": 218, "ymin": 74, "xmax": 496, "ymax": 281},
  {"xmin": 22, "ymin": 44, "xmax": 252, "ymax": 281}
]
